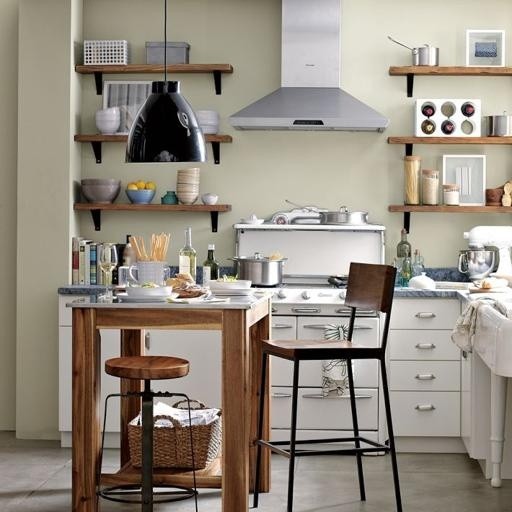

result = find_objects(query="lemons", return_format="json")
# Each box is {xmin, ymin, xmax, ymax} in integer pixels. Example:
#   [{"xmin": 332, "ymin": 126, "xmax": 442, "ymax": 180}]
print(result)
[
  {"xmin": 136, "ymin": 180, "xmax": 145, "ymax": 189},
  {"xmin": 127, "ymin": 182, "xmax": 138, "ymax": 190},
  {"xmin": 146, "ymin": 182, "xmax": 156, "ymax": 189}
]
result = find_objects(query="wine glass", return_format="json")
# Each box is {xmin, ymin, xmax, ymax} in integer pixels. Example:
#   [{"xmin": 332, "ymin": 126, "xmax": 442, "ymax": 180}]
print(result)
[{"xmin": 96, "ymin": 244, "xmax": 120, "ymax": 302}]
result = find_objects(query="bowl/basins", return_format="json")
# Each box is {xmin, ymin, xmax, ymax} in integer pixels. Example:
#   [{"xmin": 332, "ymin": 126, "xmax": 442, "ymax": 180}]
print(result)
[
  {"xmin": 125, "ymin": 190, "xmax": 156, "ymax": 204},
  {"xmin": 80, "ymin": 178, "xmax": 121, "ymax": 204},
  {"xmin": 175, "ymin": 168, "xmax": 201, "ymax": 205},
  {"xmin": 200, "ymin": 196, "xmax": 220, "ymax": 205},
  {"xmin": 96, "ymin": 107, "xmax": 122, "ymax": 137}
]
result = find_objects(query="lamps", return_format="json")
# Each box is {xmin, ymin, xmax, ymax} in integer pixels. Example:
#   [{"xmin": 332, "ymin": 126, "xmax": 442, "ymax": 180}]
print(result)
[{"xmin": 123, "ymin": 0, "xmax": 206, "ymax": 162}]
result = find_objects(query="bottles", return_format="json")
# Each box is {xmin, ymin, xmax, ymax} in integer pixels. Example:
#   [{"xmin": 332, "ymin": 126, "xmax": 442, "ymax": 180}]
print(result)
[
  {"xmin": 422, "ymin": 104, "xmax": 473, "ymax": 134},
  {"xmin": 179, "ymin": 227, "xmax": 197, "ymax": 281},
  {"xmin": 123, "ymin": 234, "xmax": 136, "ymax": 279},
  {"xmin": 162, "ymin": 190, "xmax": 178, "ymax": 205},
  {"xmin": 205, "ymin": 244, "xmax": 220, "ymax": 287},
  {"xmin": 404, "ymin": 154, "xmax": 459, "ymax": 205},
  {"xmin": 388, "ymin": 230, "xmax": 425, "ymax": 288}
]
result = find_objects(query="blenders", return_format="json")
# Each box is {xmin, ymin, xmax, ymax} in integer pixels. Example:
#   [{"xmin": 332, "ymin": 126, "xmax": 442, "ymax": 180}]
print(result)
[{"xmin": 458, "ymin": 225, "xmax": 512, "ymax": 293}]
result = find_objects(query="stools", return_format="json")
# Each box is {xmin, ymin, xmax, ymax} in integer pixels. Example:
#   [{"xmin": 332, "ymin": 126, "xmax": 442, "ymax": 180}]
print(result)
[{"xmin": 92, "ymin": 356, "xmax": 198, "ymax": 512}]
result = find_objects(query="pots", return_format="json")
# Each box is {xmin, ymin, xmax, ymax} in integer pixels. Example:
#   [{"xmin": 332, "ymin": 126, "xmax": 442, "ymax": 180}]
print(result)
[
  {"xmin": 227, "ymin": 252, "xmax": 288, "ymax": 286},
  {"xmin": 483, "ymin": 111, "xmax": 511, "ymax": 137},
  {"xmin": 386, "ymin": 36, "xmax": 438, "ymax": 66},
  {"xmin": 289, "ymin": 206, "xmax": 368, "ymax": 225}
]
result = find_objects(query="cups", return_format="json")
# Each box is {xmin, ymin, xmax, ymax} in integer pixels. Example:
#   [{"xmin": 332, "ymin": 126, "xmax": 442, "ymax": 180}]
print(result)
[
  {"xmin": 128, "ymin": 261, "xmax": 164, "ymax": 283},
  {"xmin": 119, "ymin": 267, "xmax": 130, "ymax": 287},
  {"xmin": 195, "ymin": 110, "xmax": 219, "ymax": 135},
  {"xmin": 485, "ymin": 188, "xmax": 501, "ymax": 207}
]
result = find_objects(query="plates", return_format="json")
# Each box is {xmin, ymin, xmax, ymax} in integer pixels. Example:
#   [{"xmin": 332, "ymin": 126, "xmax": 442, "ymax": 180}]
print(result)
[{"xmin": 118, "ymin": 279, "xmax": 254, "ymax": 303}]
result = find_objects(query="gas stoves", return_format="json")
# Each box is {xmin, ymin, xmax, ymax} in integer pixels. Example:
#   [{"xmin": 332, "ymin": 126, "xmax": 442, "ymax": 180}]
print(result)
[{"xmin": 251, "ymin": 274, "xmax": 348, "ymax": 305}]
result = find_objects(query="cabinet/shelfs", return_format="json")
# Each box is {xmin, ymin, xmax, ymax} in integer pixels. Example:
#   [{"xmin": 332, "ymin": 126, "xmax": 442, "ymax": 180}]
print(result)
[
  {"xmin": 461, "ymin": 296, "xmax": 512, "ymax": 483},
  {"xmin": 383, "ymin": 298, "xmax": 469, "ymax": 459},
  {"xmin": 387, "ymin": 66, "xmax": 512, "ymax": 234},
  {"xmin": 60, "ymin": 295, "xmax": 222, "ymax": 487},
  {"xmin": 266, "ymin": 315, "xmax": 383, "ymax": 457},
  {"xmin": 73, "ymin": 63, "xmax": 233, "ymax": 232}
]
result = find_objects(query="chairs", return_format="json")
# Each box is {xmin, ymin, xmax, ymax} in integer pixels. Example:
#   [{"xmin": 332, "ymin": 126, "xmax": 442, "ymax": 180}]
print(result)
[{"xmin": 254, "ymin": 261, "xmax": 405, "ymax": 512}]
